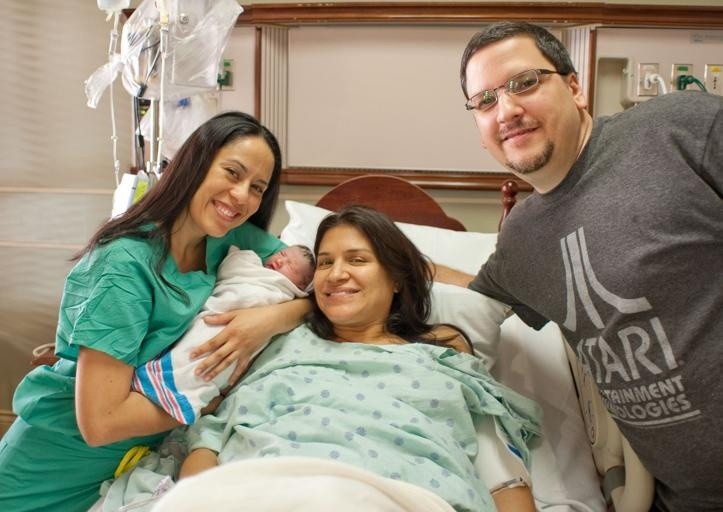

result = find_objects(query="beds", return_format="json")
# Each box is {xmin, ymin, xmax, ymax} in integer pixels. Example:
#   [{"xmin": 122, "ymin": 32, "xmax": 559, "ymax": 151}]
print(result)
[{"xmin": 74, "ymin": 172, "xmax": 654, "ymax": 511}]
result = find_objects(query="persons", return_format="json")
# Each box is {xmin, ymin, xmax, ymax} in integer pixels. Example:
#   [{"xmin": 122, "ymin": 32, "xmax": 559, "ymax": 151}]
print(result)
[
  {"xmin": 408, "ymin": 20, "xmax": 722, "ymax": 512},
  {"xmin": 0, "ymin": 114, "xmax": 311, "ymax": 512},
  {"xmin": 179, "ymin": 204, "xmax": 540, "ymax": 512},
  {"xmin": 128, "ymin": 244, "xmax": 317, "ymax": 427}
]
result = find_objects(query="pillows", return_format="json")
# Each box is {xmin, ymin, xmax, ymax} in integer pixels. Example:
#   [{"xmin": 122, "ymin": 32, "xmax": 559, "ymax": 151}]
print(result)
[{"xmin": 278, "ymin": 193, "xmax": 507, "ymax": 376}]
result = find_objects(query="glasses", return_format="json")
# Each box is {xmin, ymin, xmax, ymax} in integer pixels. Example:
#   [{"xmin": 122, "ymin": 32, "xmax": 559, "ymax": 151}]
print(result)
[{"xmin": 465, "ymin": 68, "xmax": 560, "ymax": 113}]
